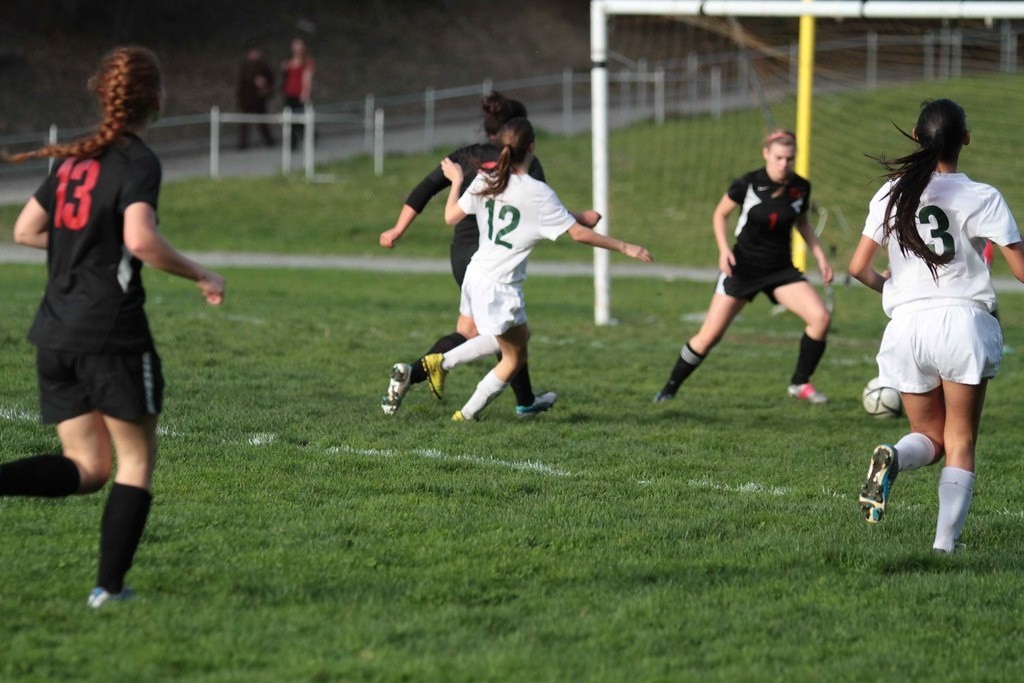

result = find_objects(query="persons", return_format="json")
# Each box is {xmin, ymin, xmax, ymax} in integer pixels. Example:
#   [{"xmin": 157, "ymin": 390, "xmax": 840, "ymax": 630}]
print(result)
[
  {"xmin": 1, "ymin": 48, "xmax": 224, "ymax": 609},
  {"xmin": 848, "ymin": 98, "xmax": 1024, "ymax": 552},
  {"xmin": 657, "ymin": 131, "xmax": 835, "ymax": 404},
  {"xmin": 380, "ymin": 90, "xmax": 600, "ymax": 414},
  {"xmin": 237, "ymin": 36, "xmax": 317, "ymax": 152},
  {"xmin": 421, "ymin": 117, "xmax": 655, "ymax": 421}
]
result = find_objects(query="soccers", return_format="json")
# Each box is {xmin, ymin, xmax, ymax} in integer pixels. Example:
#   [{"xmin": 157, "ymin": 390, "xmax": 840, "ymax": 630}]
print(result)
[{"xmin": 861, "ymin": 376, "xmax": 904, "ymax": 419}]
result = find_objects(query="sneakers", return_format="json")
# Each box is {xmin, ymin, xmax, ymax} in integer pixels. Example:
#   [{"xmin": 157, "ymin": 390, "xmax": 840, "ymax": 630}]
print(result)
[
  {"xmin": 515, "ymin": 392, "xmax": 557, "ymax": 418},
  {"xmin": 453, "ymin": 411, "xmax": 464, "ymax": 421},
  {"xmin": 88, "ymin": 587, "xmax": 133, "ymax": 608},
  {"xmin": 380, "ymin": 362, "xmax": 411, "ymax": 416},
  {"xmin": 859, "ymin": 444, "xmax": 899, "ymax": 523},
  {"xmin": 929, "ymin": 545, "xmax": 958, "ymax": 558},
  {"xmin": 790, "ymin": 382, "xmax": 828, "ymax": 403},
  {"xmin": 420, "ymin": 353, "xmax": 448, "ymax": 400}
]
